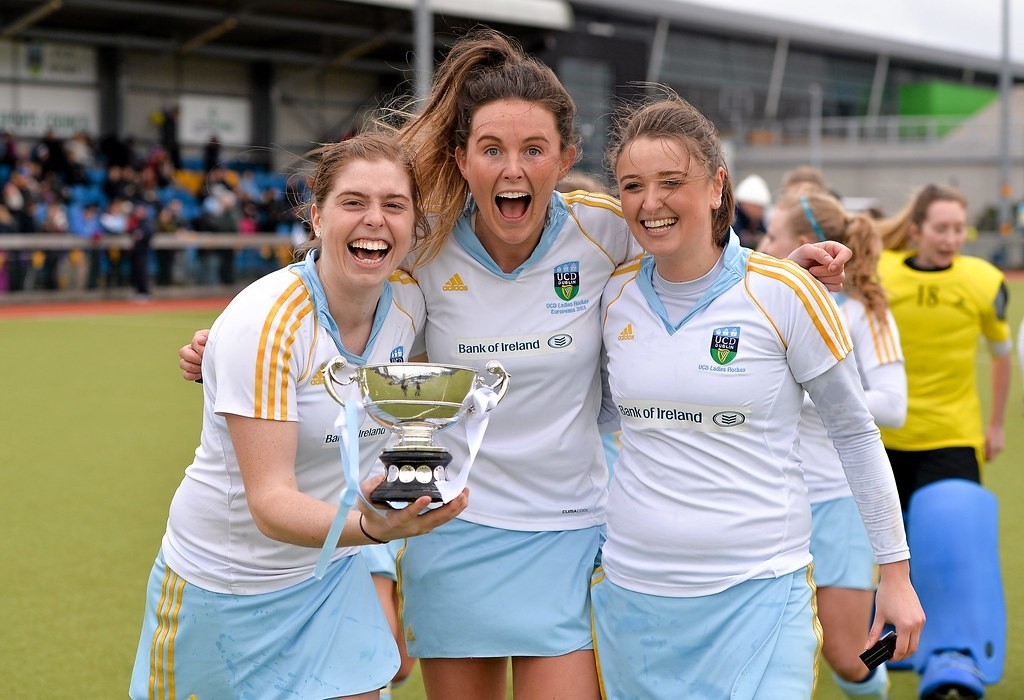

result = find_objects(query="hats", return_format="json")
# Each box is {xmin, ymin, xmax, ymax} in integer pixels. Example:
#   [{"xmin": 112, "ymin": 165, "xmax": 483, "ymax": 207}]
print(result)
[{"xmin": 734, "ymin": 174, "xmax": 772, "ymax": 208}]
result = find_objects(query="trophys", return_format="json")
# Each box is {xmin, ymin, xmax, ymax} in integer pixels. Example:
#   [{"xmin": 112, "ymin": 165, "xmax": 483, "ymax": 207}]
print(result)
[{"xmin": 322, "ymin": 355, "xmax": 511, "ymax": 503}]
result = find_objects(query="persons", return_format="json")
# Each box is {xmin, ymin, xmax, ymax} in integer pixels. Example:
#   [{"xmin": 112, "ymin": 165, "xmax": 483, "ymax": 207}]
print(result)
[
  {"xmin": 415, "ymin": 376, "xmax": 423, "ymax": 394},
  {"xmin": 400, "ymin": 372, "xmax": 408, "ymax": 395},
  {"xmin": 0, "ymin": 31, "xmax": 1024, "ymax": 700}
]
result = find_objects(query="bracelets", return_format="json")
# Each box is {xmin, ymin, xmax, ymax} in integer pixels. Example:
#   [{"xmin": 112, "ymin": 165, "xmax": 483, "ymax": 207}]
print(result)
[{"xmin": 360, "ymin": 514, "xmax": 389, "ymax": 544}]
